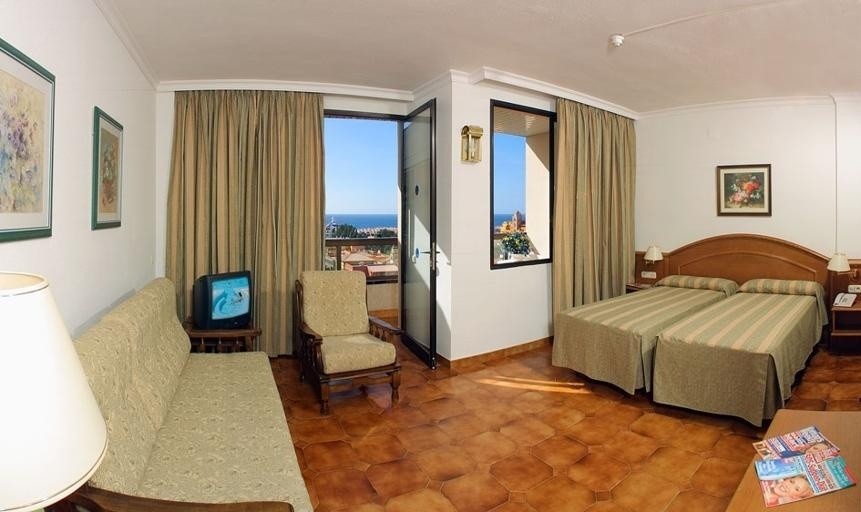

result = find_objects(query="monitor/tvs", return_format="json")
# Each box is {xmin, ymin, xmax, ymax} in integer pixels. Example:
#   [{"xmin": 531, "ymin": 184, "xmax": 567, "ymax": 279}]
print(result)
[{"xmin": 193, "ymin": 271, "xmax": 253, "ymax": 331}]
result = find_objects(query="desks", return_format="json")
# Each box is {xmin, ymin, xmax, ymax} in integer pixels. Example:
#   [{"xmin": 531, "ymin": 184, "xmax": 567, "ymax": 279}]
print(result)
[
  {"xmin": 181, "ymin": 325, "xmax": 265, "ymax": 354},
  {"xmin": 723, "ymin": 409, "xmax": 861, "ymax": 512}
]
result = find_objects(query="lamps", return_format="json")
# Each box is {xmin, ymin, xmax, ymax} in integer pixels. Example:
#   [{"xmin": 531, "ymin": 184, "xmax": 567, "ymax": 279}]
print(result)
[
  {"xmin": 0, "ymin": 271, "xmax": 110, "ymax": 512},
  {"xmin": 643, "ymin": 244, "xmax": 665, "ymax": 265},
  {"xmin": 827, "ymin": 251, "xmax": 859, "ymax": 282},
  {"xmin": 462, "ymin": 123, "xmax": 486, "ymax": 165}
]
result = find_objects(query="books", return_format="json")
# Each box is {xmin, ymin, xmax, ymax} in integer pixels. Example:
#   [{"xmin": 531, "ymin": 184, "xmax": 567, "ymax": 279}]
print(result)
[
  {"xmin": 753, "ymin": 424, "xmax": 841, "ymax": 461},
  {"xmin": 754, "ymin": 447, "xmax": 858, "ymax": 507}
]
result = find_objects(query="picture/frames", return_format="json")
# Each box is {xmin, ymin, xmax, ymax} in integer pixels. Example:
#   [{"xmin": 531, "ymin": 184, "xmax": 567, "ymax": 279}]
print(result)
[
  {"xmin": 716, "ymin": 159, "xmax": 772, "ymax": 219},
  {"xmin": 0, "ymin": 38, "xmax": 57, "ymax": 243},
  {"xmin": 89, "ymin": 106, "xmax": 127, "ymax": 232}
]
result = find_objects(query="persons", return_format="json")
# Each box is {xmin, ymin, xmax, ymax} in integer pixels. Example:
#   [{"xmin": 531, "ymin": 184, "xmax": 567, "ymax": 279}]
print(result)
[
  {"xmin": 762, "ymin": 473, "xmax": 814, "ymax": 505},
  {"xmin": 780, "ymin": 441, "xmax": 829, "ymax": 459}
]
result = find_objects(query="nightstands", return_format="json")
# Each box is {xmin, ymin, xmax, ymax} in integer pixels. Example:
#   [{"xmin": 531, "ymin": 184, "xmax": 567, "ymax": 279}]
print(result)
[
  {"xmin": 626, "ymin": 281, "xmax": 650, "ymax": 292},
  {"xmin": 829, "ymin": 300, "xmax": 861, "ymax": 351}
]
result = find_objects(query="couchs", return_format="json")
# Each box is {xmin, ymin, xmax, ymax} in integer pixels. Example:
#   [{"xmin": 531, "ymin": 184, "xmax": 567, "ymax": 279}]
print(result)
[{"xmin": 66, "ymin": 271, "xmax": 317, "ymax": 512}]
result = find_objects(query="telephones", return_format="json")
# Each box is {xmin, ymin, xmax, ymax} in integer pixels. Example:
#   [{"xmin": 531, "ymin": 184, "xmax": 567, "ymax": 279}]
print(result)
[{"xmin": 832, "ymin": 293, "xmax": 858, "ymax": 307}]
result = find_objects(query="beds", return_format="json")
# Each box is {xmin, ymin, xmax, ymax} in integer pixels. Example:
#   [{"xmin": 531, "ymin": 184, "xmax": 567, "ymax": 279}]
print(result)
[
  {"xmin": 652, "ymin": 278, "xmax": 826, "ymax": 427},
  {"xmin": 551, "ymin": 276, "xmax": 741, "ymax": 395}
]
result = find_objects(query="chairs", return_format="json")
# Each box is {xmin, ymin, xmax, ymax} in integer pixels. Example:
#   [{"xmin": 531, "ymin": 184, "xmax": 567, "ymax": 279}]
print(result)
[{"xmin": 291, "ymin": 267, "xmax": 405, "ymax": 419}]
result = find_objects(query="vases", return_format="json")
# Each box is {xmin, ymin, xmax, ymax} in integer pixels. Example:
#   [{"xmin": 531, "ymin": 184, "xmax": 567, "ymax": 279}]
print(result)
[{"xmin": 503, "ymin": 251, "xmax": 524, "ymax": 261}]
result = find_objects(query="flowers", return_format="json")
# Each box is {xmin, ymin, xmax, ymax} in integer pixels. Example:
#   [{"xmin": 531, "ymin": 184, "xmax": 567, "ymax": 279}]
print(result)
[{"xmin": 498, "ymin": 223, "xmax": 532, "ymax": 253}]
